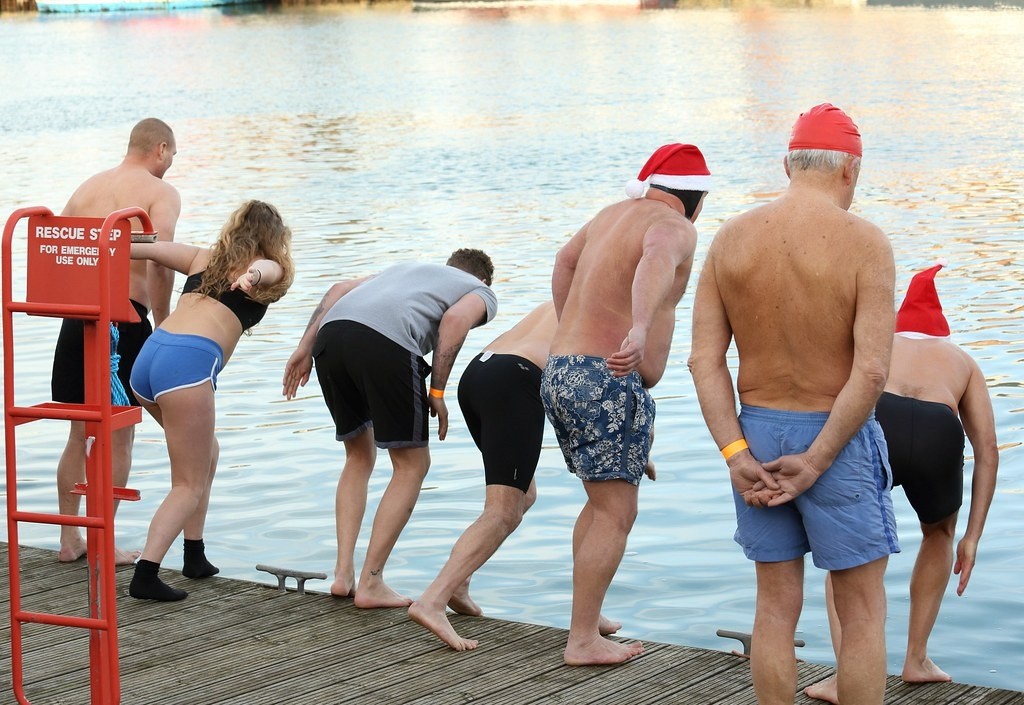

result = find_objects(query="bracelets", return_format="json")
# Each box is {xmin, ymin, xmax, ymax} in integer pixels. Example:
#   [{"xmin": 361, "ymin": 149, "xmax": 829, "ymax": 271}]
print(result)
[
  {"xmin": 720, "ymin": 438, "xmax": 749, "ymax": 461},
  {"xmin": 252, "ymin": 268, "xmax": 261, "ymax": 286},
  {"xmin": 429, "ymin": 385, "xmax": 444, "ymax": 399}
]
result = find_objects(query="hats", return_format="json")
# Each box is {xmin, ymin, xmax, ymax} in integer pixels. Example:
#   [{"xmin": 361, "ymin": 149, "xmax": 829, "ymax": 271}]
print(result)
[
  {"xmin": 894, "ymin": 257, "xmax": 951, "ymax": 339},
  {"xmin": 625, "ymin": 143, "xmax": 712, "ymax": 199},
  {"xmin": 789, "ymin": 103, "xmax": 863, "ymax": 158}
]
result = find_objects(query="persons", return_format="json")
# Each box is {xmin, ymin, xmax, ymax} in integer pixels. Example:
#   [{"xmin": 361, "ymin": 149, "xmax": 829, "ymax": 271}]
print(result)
[
  {"xmin": 801, "ymin": 264, "xmax": 1000, "ymax": 705},
  {"xmin": 129, "ymin": 199, "xmax": 295, "ymax": 602},
  {"xmin": 56, "ymin": 117, "xmax": 182, "ymax": 566},
  {"xmin": 686, "ymin": 102, "xmax": 902, "ymax": 705},
  {"xmin": 539, "ymin": 142, "xmax": 712, "ymax": 665},
  {"xmin": 281, "ymin": 248, "xmax": 499, "ymax": 608},
  {"xmin": 407, "ymin": 298, "xmax": 657, "ymax": 652}
]
